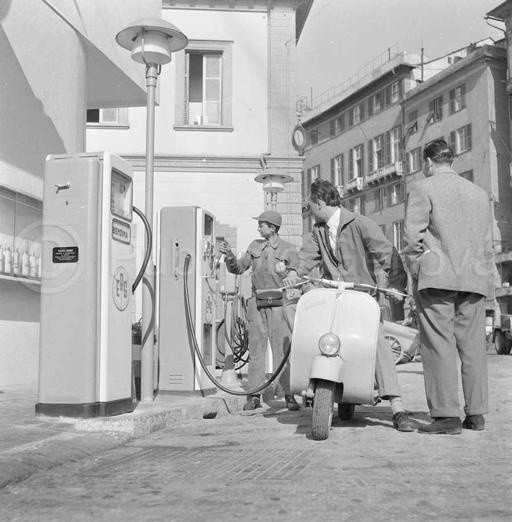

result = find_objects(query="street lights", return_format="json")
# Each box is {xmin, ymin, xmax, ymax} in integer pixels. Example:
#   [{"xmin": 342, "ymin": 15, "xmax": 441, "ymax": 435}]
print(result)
[
  {"xmin": 254, "ymin": 166, "xmax": 294, "ymax": 238},
  {"xmin": 111, "ymin": 17, "xmax": 193, "ymax": 405}
]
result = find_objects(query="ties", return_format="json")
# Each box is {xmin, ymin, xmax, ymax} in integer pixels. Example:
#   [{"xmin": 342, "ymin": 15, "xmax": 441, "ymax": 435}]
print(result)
[{"xmin": 324, "ymin": 223, "xmax": 339, "ymax": 266}]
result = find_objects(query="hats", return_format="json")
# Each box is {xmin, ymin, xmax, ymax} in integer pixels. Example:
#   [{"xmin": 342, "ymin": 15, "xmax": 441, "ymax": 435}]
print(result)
[{"xmin": 252, "ymin": 211, "xmax": 282, "ymax": 226}]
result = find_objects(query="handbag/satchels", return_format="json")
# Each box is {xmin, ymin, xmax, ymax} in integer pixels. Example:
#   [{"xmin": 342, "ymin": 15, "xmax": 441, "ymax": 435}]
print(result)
[{"xmin": 255, "ymin": 289, "xmax": 284, "ymax": 307}]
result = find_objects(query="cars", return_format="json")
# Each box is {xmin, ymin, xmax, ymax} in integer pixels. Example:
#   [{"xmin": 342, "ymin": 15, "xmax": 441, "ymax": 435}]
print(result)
[{"xmin": 491, "ymin": 313, "xmax": 512, "ymax": 357}]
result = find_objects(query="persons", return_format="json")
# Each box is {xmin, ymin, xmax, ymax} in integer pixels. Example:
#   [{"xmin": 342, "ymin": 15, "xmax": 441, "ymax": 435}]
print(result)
[
  {"xmin": 219, "ymin": 209, "xmax": 299, "ymax": 411},
  {"xmin": 282, "ymin": 177, "xmax": 418, "ymax": 432},
  {"xmin": 402, "ymin": 137, "xmax": 502, "ymax": 436}
]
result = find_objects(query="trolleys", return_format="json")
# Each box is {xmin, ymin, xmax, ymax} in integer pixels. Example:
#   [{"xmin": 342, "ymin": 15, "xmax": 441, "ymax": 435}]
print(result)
[{"xmin": 380, "ymin": 305, "xmax": 422, "ymax": 365}]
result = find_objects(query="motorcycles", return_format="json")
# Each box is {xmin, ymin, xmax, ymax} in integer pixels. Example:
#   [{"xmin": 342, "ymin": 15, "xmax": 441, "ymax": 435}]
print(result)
[{"xmin": 274, "ymin": 259, "xmax": 413, "ymax": 441}]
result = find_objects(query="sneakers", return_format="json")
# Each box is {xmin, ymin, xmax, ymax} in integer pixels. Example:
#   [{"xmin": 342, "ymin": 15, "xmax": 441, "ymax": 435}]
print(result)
[
  {"xmin": 418, "ymin": 417, "xmax": 462, "ymax": 434},
  {"xmin": 243, "ymin": 396, "xmax": 260, "ymax": 410},
  {"xmin": 285, "ymin": 397, "xmax": 299, "ymax": 411},
  {"xmin": 463, "ymin": 415, "xmax": 484, "ymax": 430},
  {"xmin": 392, "ymin": 412, "xmax": 414, "ymax": 431}
]
